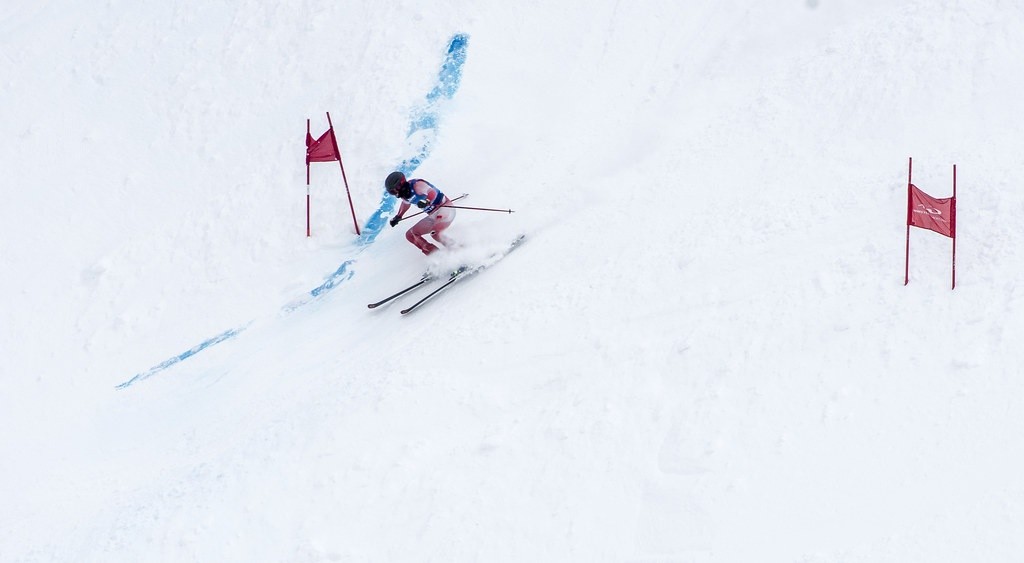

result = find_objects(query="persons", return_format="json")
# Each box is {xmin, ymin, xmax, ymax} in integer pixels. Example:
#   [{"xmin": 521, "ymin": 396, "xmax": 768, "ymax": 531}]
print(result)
[{"xmin": 382, "ymin": 170, "xmax": 468, "ymax": 277}]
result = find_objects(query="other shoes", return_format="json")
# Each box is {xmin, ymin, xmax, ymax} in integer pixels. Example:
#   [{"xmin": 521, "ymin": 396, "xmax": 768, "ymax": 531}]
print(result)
[
  {"xmin": 450, "ymin": 266, "xmax": 466, "ymax": 278},
  {"xmin": 421, "ymin": 269, "xmax": 432, "ymax": 278}
]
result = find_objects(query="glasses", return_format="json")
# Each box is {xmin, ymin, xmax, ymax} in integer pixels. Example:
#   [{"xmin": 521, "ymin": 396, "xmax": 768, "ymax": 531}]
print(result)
[{"xmin": 387, "ymin": 189, "xmax": 398, "ymax": 195}]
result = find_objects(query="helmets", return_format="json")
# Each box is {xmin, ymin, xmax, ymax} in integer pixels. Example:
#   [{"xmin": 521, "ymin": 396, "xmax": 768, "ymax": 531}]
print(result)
[{"xmin": 385, "ymin": 172, "xmax": 408, "ymax": 197}]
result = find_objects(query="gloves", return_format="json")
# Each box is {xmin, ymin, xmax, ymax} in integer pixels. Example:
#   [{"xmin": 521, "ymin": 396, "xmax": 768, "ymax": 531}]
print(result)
[
  {"xmin": 417, "ymin": 201, "xmax": 426, "ymax": 209},
  {"xmin": 390, "ymin": 218, "xmax": 398, "ymax": 227}
]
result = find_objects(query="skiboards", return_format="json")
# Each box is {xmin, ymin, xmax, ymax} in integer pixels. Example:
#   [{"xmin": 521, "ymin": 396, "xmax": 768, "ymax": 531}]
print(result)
[{"xmin": 367, "ymin": 232, "xmax": 527, "ymax": 316}]
